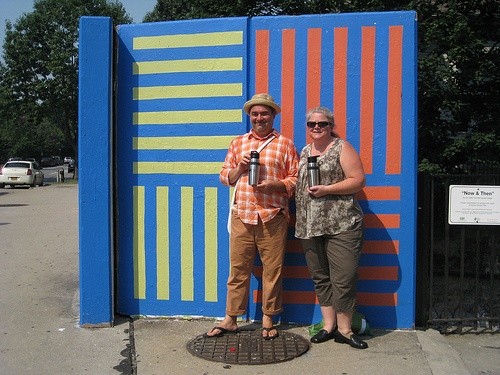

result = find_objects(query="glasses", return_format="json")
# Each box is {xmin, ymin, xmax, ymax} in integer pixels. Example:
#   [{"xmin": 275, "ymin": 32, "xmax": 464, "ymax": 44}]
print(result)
[{"xmin": 306, "ymin": 121, "xmax": 332, "ymax": 128}]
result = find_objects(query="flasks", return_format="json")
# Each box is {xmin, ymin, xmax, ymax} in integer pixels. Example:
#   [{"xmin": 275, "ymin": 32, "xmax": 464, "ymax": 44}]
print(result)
[
  {"xmin": 249, "ymin": 150, "xmax": 260, "ymax": 185},
  {"xmin": 308, "ymin": 154, "xmax": 321, "ymax": 187}
]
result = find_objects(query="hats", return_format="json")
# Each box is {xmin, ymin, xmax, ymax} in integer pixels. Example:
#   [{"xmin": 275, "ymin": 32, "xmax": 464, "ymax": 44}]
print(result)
[{"xmin": 243, "ymin": 94, "xmax": 281, "ymax": 116}]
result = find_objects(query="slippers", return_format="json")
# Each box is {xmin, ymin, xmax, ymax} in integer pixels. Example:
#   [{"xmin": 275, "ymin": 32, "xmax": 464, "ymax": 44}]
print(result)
[
  {"xmin": 261, "ymin": 327, "xmax": 279, "ymax": 339},
  {"xmin": 203, "ymin": 327, "xmax": 240, "ymax": 338}
]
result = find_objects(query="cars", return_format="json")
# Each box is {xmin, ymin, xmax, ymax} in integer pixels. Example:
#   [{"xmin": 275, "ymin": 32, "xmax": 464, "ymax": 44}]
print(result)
[
  {"xmin": 0, "ymin": 160, "xmax": 44, "ymax": 188},
  {"xmin": 64, "ymin": 157, "xmax": 71, "ymax": 163},
  {"xmin": 67, "ymin": 159, "xmax": 75, "ymax": 173},
  {"xmin": 25, "ymin": 158, "xmax": 41, "ymax": 170}
]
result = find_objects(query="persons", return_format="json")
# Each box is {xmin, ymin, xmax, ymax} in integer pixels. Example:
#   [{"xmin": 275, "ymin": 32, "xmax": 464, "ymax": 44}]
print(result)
[
  {"xmin": 203, "ymin": 93, "xmax": 300, "ymax": 340},
  {"xmin": 295, "ymin": 107, "xmax": 369, "ymax": 349}
]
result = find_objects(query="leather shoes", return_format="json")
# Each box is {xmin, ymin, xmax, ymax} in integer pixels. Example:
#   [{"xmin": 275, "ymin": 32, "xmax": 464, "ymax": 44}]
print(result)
[
  {"xmin": 334, "ymin": 330, "xmax": 368, "ymax": 349},
  {"xmin": 310, "ymin": 326, "xmax": 338, "ymax": 343}
]
